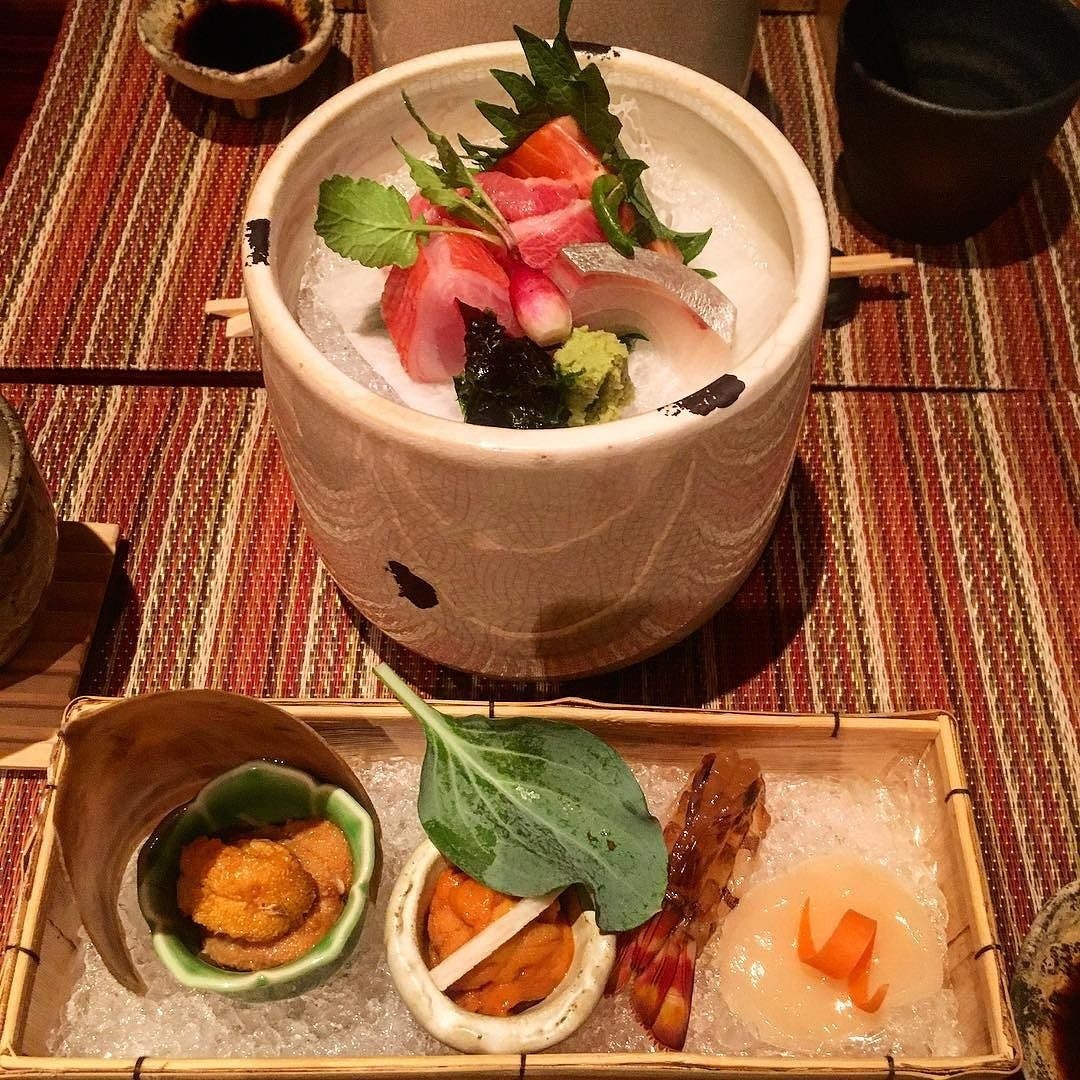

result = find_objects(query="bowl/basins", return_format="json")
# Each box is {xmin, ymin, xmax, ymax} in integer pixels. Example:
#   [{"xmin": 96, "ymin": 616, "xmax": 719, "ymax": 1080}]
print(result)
[
  {"xmin": 134, "ymin": 0, "xmax": 337, "ymax": 120},
  {"xmin": 242, "ymin": 39, "xmax": 832, "ymax": 680},
  {"xmin": 137, "ymin": 759, "xmax": 374, "ymax": 991}
]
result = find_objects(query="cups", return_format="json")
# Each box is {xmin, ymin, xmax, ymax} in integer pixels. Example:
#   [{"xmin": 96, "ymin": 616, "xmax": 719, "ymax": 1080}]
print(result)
[
  {"xmin": 836, "ymin": 0, "xmax": 1079, "ymax": 246},
  {"xmin": 0, "ymin": 395, "xmax": 59, "ymax": 660}
]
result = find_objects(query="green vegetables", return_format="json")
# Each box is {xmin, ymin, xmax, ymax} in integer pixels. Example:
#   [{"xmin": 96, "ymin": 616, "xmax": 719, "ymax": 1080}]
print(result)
[
  {"xmin": 373, "ymin": 662, "xmax": 667, "ymax": 931},
  {"xmin": 315, "ymin": 0, "xmax": 717, "ymax": 279}
]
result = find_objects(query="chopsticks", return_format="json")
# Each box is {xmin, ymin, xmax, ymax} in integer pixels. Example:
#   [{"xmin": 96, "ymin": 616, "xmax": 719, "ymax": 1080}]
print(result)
[{"xmin": 205, "ymin": 254, "xmax": 915, "ymax": 337}]
